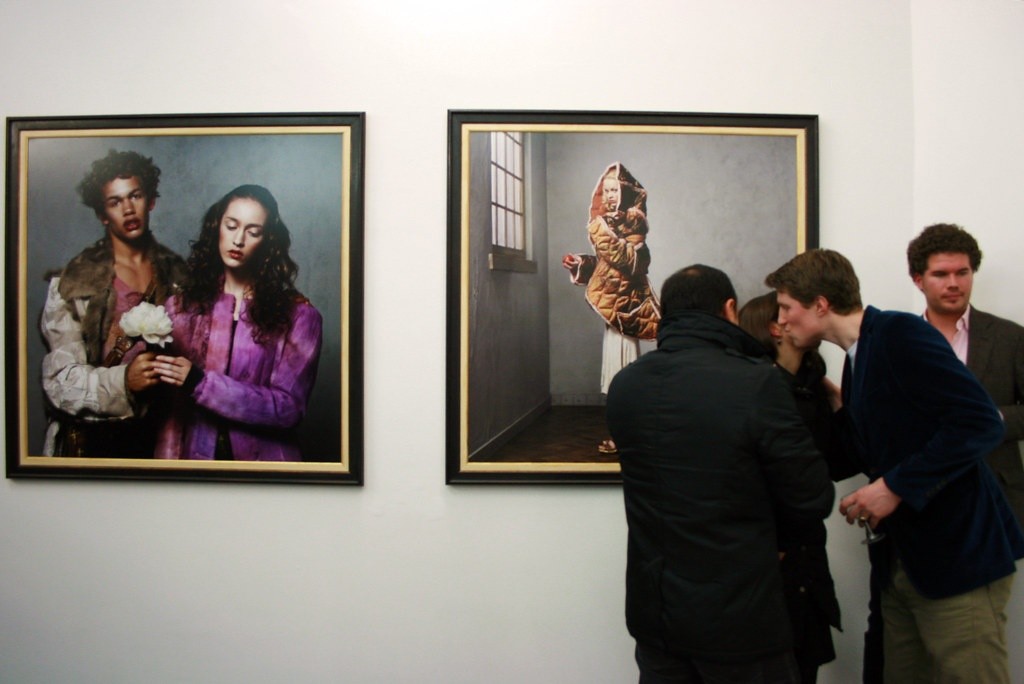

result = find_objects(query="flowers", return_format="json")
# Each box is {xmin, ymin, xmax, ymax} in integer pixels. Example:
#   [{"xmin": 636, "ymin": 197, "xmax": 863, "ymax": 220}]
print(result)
[{"xmin": 120, "ymin": 301, "xmax": 175, "ymax": 352}]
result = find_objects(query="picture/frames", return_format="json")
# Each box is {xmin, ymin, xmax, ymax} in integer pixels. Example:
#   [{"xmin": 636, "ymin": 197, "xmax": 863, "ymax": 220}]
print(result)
[
  {"xmin": 6, "ymin": 111, "xmax": 365, "ymax": 486},
  {"xmin": 445, "ymin": 106, "xmax": 819, "ymax": 489}
]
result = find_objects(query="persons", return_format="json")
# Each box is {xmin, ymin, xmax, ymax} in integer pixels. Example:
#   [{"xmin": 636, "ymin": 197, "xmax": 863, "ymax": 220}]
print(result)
[
  {"xmin": 605, "ymin": 264, "xmax": 836, "ymax": 684},
  {"xmin": 738, "ymin": 290, "xmax": 845, "ymax": 634},
  {"xmin": 153, "ymin": 185, "xmax": 322, "ymax": 461},
  {"xmin": 907, "ymin": 223, "xmax": 1024, "ymax": 523},
  {"xmin": 563, "ymin": 162, "xmax": 661, "ymax": 452},
  {"xmin": 42, "ymin": 149, "xmax": 184, "ymax": 458},
  {"xmin": 765, "ymin": 249, "xmax": 1024, "ymax": 684}
]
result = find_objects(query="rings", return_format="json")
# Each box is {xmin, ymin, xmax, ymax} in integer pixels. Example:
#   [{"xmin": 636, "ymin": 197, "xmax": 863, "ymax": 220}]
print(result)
[{"xmin": 860, "ymin": 517, "xmax": 866, "ymax": 521}]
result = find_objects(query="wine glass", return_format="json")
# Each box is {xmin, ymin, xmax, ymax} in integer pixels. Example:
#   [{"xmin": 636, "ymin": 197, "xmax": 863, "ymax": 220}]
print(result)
[{"xmin": 839, "ymin": 487, "xmax": 885, "ymax": 543}]
